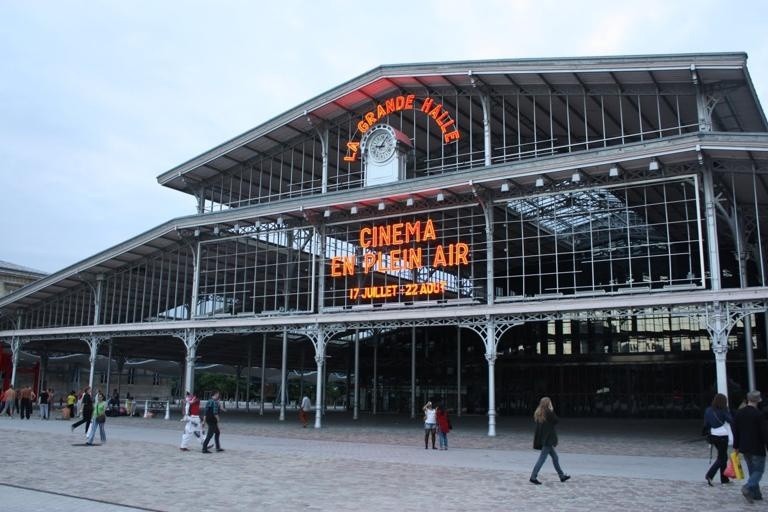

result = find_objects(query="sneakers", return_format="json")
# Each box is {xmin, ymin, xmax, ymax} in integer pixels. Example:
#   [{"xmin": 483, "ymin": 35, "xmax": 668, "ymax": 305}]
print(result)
[
  {"xmin": 561, "ymin": 476, "xmax": 570, "ymax": 482},
  {"xmin": 203, "ymin": 444, "xmax": 224, "ymax": 453},
  {"xmin": 706, "ymin": 476, "xmax": 713, "ymax": 486},
  {"xmin": 530, "ymin": 479, "xmax": 541, "ymax": 484}
]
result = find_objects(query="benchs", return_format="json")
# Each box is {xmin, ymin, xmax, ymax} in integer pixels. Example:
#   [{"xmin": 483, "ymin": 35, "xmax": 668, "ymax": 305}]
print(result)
[
  {"xmin": 108, "ymin": 398, "xmax": 136, "ymax": 417},
  {"xmin": 142, "ymin": 399, "xmax": 170, "ymax": 421}
]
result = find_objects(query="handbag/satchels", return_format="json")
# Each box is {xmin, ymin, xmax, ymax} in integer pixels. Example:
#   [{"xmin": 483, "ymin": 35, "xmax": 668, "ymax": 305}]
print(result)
[
  {"xmin": 206, "ymin": 407, "xmax": 217, "ymax": 424},
  {"xmin": 96, "ymin": 416, "xmax": 105, "ymax": 423},
  {"xmin": 723, "ymin": 452, "xmax": 744, "ymax": 480}
]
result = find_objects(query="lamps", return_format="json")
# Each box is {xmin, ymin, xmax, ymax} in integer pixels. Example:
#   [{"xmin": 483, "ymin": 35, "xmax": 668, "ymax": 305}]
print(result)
[
  {"xmin": 607, "ymin": 166, "xmax": 621, "ymax": 178},
  {"xmin": 277, "ymin": 214, "xmax": 286, "ymax": 227},
  {"xmin": 350, "ymin": 205, "xmax": 358, "ymax": 214},
  {"xmin": 230, "ymin": 224, "xmax": 240, "ymax": 233},
  {"xmin": 435, "ymin": 190, "xmax": 445, "ymax": 201},
  {"xmin": 192, "ymin": 226, "xmax": 201, "ymax": 237},
  {"xmin": 212, "ymin": 225, "xmax": 221, "ymax": 235},
  {"xmin": 377, "ymin": 201, "xmax": 386, "ymax": 211},
  {"xmin": 324, "ymin": 209, "xmax": 335, "ymax": 219},
  {"xmin": 536, "ymin": 176, "xmax": 545, "ymax": 187},
  {"xmin": 572, "ymin": 172, "xmax": 581, "ymax": 183},
  {"xmin": 407, "ymin": 197, "xmax": 414, "ymax": 207},
  {"xmin": 500, "ymin": 181, "xmax": 510, "ymax": 192},
  {"xmin": 254, "ymin": 219, "xmax": 262, "ymax": 227},
  {"xmin": 648, "ymin": 160, "xmax": 660, "ymax": 173}
]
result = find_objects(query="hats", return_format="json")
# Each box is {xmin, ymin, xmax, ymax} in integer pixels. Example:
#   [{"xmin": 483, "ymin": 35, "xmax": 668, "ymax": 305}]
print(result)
[{"xmin": 747, "ymin": 390, "xmax": 762, "ymax": 402}]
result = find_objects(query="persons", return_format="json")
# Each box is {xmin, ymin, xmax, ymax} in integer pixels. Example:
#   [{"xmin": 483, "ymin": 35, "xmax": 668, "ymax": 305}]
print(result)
[
  {"xmin": 703, "ymin": 393, "xmax": 734, "ymax": 486},
  {"xmin": 732, "ymin": 390, "xmax": 767, "ymax": 504},
  {"xmin": 527, "ymin": 396, "xmax": 570, "ymax": 485},
  {"xmin": 434, "ymin": 402, "xmax": 451, "ymax": 451},
  {"xmin": 298, "ymin": 393, "xmax": 311, "ymax": 427},
  {"xmin": 0, "ymin": 382, "xmax": 226, "ymax": 454},
  {"xmin": 422, "ymin": 400, "xmax": 436, "ymax": 451}
]
